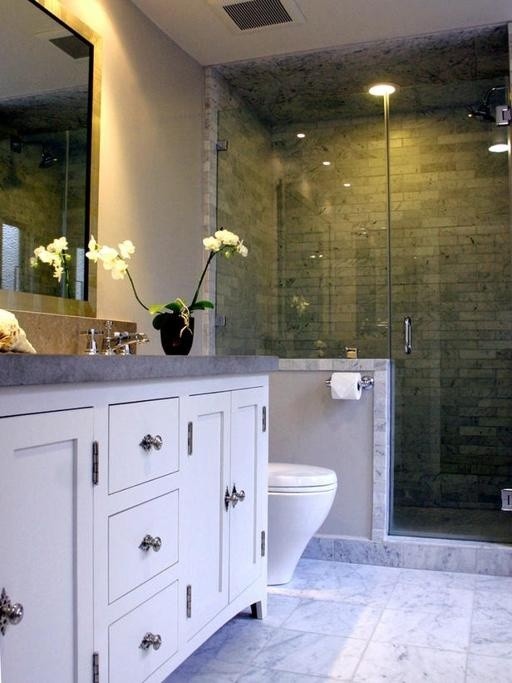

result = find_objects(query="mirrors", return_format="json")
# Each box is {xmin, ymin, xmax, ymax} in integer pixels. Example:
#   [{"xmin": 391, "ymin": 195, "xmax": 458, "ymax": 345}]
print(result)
[{"xmin": 0, "ymin": 0, "xmax": 103, "ymax": 321}]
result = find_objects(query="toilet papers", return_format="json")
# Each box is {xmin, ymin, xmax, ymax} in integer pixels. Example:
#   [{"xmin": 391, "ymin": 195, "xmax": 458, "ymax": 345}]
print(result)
[{"xmin": 329, "ymin": 371, "xmax": 362, "ymax": 400}]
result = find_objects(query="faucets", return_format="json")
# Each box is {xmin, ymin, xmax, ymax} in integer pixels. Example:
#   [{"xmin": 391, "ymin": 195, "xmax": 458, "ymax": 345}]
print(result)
[{"xmin": 102, "ymin": 328, "xmax": 151, "ymax": 353}]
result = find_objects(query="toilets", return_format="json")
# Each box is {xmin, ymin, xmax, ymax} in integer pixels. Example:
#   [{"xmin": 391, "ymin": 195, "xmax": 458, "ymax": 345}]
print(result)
[{"xmin": 267, "ymin": 460, "xmax": 338, "ymax": 585}]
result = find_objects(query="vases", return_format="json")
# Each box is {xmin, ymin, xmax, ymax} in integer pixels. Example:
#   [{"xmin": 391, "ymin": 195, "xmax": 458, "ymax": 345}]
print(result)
[{"xmin": 152, "ymin": 313, "xmax": 196, "ymax": 355}]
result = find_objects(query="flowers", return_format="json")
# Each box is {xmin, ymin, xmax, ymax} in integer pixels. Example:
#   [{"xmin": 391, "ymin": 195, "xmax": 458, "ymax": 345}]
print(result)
[
  {"xmin": 87, "ymin": 227, "xmax": 250, "ymax": 315},
  {"xmin": 31, "ymin": 237, "xmax": 75, "ymax": 299}
]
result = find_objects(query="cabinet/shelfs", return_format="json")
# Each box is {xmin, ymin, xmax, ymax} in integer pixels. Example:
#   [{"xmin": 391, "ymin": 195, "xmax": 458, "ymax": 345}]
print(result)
[
  {"xmin": 179, "ymin": 375, "xmax": 269, "ymax": 663},
  {"xmin": 0, "ymin": 379, "xmax": 180, "ymax": 683}
]
